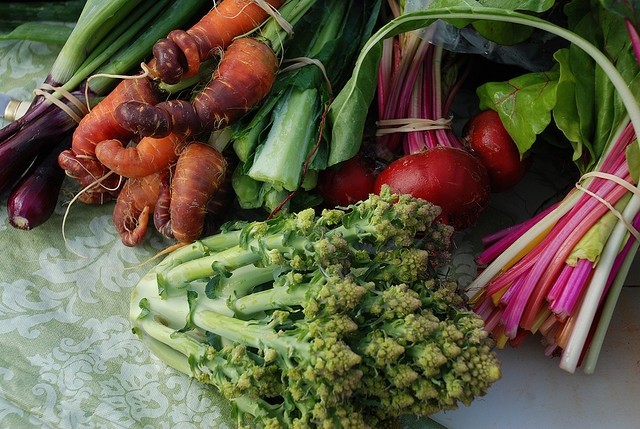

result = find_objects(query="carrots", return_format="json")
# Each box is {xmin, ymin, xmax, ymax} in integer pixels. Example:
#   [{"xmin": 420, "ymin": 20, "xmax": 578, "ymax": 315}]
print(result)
[{"xmin": 57, "ymin": 1, "xmax": 280, "ymax": 269}]
